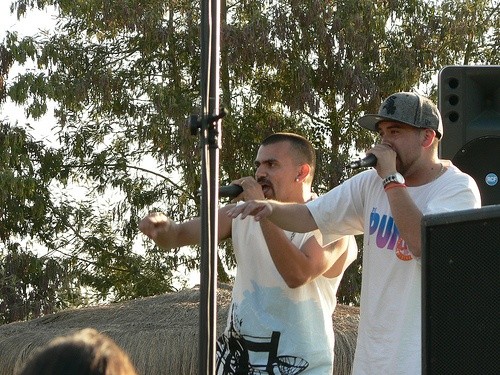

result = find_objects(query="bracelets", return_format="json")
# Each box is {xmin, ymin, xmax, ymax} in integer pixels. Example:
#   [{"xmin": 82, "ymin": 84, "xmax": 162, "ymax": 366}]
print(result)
[{"xmin": 384, "ymin": 182, "xmax": 407, "ymax": 192}]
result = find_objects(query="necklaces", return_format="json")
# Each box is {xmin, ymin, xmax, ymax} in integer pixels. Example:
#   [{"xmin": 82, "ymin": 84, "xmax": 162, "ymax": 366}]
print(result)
[{"xmin": 431, "ymin": 162, "xmax": 443, "ymax": 181}]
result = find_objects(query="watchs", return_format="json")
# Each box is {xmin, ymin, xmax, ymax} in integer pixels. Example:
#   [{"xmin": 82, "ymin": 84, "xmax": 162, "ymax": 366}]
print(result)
[{"xmin": 382, "ymin": 172, "xmax": 405, "ymax": 189}]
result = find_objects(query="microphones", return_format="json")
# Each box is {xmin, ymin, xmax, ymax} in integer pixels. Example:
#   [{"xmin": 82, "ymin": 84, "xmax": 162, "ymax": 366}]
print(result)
[
  {"xmin": 345, "ymin": 153, "xmax": 377, "ymax": 169},
  {"xmin": 218, "ymin": 185, "xmax": 243, "ymax": 197}
]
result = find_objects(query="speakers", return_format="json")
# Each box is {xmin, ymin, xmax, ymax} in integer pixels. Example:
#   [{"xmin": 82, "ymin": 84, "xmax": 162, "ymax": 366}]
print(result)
[
  {"xmin": 420, "ymin": 206, "xmax": 500, "ymax": 375},
  {"xmin": 437, "ymin": 65, "xmax": 500, "ymax": 205}
]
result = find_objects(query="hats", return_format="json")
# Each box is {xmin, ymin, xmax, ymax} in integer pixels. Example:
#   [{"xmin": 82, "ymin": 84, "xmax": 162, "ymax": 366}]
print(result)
[{"xmin": 358, "ymin": 92, "xmax": 444, "ymax": 141}]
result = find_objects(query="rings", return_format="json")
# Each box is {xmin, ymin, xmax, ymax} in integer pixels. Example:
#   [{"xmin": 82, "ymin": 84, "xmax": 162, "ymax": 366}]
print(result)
[{"xmin": 241, "ymin": 180, "xmax": 245, "ymax": 184}]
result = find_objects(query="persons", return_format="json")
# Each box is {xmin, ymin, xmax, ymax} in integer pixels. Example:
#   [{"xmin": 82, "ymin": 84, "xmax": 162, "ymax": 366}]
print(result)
[
  {"xmin": 137, "ymin": 133, "xmax": 357, "ymax": 375},
  {"xmin": 225, "ymin": 92, "xmax": 481, "ymax": 375},
  {"xmin": 15, "ymin": 327, "xmax": 137, "ymax": 375}
]
